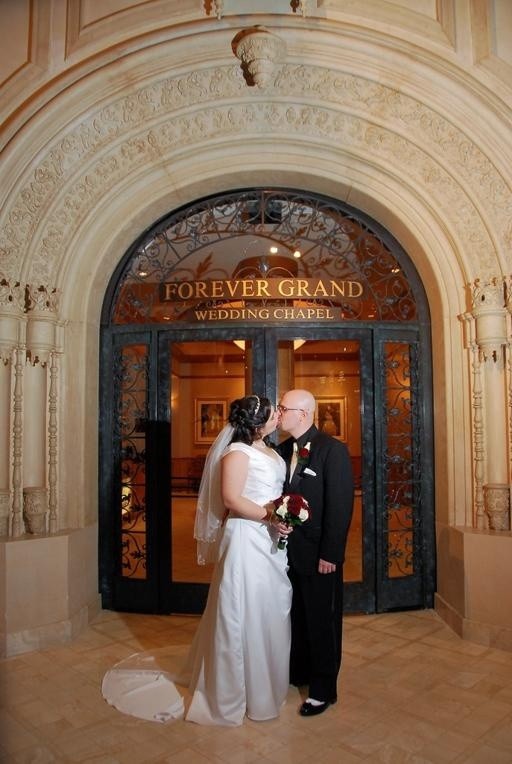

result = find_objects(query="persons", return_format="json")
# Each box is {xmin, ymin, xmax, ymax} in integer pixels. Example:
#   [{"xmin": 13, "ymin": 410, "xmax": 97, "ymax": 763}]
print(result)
[
  {"xmin": 320, "ymin": 404, "xmax": 339, "ymax": 435},
  {"xmin": 275, "ymin": 387, "xmax": 356, "ymax": 716},
  {"xmin": 204, "ymin": 394, "xmax": 293, "ymax": 721}
]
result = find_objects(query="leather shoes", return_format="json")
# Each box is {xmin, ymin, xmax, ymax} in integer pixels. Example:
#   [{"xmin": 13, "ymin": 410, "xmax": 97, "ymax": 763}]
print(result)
[{"xmin": 300, "ymin": 696, "xmax": 337, "ymax": 716}]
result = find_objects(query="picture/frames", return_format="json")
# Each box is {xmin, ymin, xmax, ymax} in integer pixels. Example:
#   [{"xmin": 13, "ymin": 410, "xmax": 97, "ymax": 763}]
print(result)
[
  {"xmin": 192, "ymin": 394, "xmax": 231, "ymax": 447},
  {"xmin": 313, "ymin": 395, "xmax": 348, "ymax": 445}
]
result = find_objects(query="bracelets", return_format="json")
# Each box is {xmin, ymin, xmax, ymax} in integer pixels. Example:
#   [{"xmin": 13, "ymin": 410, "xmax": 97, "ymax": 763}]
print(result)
[{"xmin": 268, "ymin": 508, "xmax": 275, "ymax": 526}]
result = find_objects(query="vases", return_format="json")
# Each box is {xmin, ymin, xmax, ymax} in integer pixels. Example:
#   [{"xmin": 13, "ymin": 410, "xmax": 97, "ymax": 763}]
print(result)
[
  {"xmin": 23, "ymin": 486, "xmax": 48, "ymax": 536},
  {"xmin": 483, "ymin": 483, "xmax": 510, "ymax": 530}
]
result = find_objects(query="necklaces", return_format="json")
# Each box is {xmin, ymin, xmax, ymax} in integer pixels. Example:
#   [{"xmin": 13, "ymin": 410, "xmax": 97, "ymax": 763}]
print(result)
[{"xmin": 252, "ymin": 440, "xmax": 266, "ymax": 448}]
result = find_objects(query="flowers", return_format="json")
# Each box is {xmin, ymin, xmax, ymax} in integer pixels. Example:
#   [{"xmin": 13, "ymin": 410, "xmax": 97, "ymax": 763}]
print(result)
[
  {"xmin": 272, "ymin": 493, "xmax": 311, "ymax": 549},
  {"xmin": 299, "ymin": 448, "xmax": 310, "ymax": 465}
]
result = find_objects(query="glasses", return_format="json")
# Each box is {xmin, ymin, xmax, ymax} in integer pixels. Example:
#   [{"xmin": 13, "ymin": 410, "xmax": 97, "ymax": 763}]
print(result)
[{"xmin": 277, "ymin": 405, "xmax": 308, "ymax": 416}]
